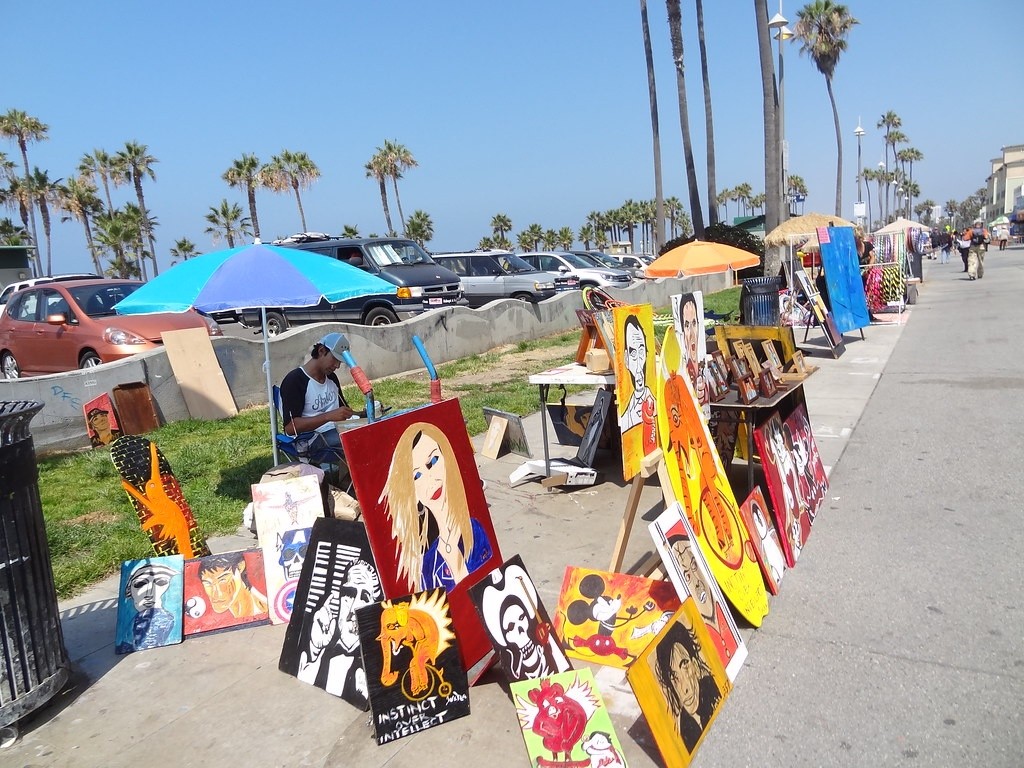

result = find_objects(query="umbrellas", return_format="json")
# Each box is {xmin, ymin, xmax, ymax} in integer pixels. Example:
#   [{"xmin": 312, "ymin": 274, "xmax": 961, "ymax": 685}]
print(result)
[
  {"xmin": 763, "ymin": 213, "xmax": 859, "ymax": 278},
  {"xmin": 988, "ymin": 214, "xmax": 1009, "ymax": 229},
  {"xmin": 111, "ymin": 236, "xmax": 400, "ymax": 464},
  {"xmin": 872, "ymin": 215, "xmax": 932, "ymax": 237},
  {"xmin": 1010, "ymin": 208, "xmax": 1024, "ymax": 235},
  {"xmin": 642, "ymin": 238, "xmax": 761, "ymax": 289}
]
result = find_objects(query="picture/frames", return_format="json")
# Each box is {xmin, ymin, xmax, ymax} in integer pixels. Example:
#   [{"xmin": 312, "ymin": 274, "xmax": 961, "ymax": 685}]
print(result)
[
  {"xmin": 742, "ymin": 342, "xmax": 762, "ymax": 379},
  {"xmin": 707, "ymin": 366, "xmax": 725, "ymax": 402},
  {"xmin": 792, "ymin": 350, "xmax": 808, "ymax": 373},
  {"xmin": 726, "ymin": 353, "xmax": 742, "ymax": 379},
  {"xmin": 711, "ymin": 350, "xmax": 733, "ymax": 384},
  {"xmin": 709, "ymin": 359, "xmax": 731, "ymax": 393},
  {"xmin": 736, "ymin": 372, "xmax": 757, "ymax": 404},
  {"xmin": 761, "ymin": 339, "xmax": 783, "ymax": 369},
  {"xmin": 758, "ymin": 368, "xmax": 778, "ymax": 398},
  {"xmin": 733, "ymin": 339, "xmax": 746, "ymax": 357},
  {"xmin": 736, "ymin": 356, "xmax": 755, "ymax": 379}
]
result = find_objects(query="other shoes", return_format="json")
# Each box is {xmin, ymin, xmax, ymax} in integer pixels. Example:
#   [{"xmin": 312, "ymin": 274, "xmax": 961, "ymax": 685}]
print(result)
[{"xmin": 970, "ymin": 278, "xmax": 976, "ymax": 280}]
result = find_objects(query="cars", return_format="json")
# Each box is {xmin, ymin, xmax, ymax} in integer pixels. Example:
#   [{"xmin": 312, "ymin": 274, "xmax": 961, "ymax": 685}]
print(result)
[{"xmin": 0, "ymin": 272, "xmax": 239, "ymax": 381}]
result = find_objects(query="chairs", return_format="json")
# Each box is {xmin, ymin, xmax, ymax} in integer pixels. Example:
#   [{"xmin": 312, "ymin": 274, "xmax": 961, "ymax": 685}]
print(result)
[{"xmin": 273, "ymin": 386, "xmax": 393, "ymax": 467}]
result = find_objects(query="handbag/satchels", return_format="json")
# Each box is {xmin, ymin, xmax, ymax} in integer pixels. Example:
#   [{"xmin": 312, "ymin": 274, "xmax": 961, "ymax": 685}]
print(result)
[{"xmin": 960, "ymin": 237, "xmax": 971, "ymax": 248}]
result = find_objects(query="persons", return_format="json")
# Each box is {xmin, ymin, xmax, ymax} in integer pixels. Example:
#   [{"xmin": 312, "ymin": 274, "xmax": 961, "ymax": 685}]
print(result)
[
  {"xmin": 928, "ymin": 224, "xmax": 958, "ymax": 265},
  {"xmin": 852, "ymin": 224, "xmax": 876, "ymax": 288},
  {"xmin": 279, "ymin": 332, "xmax": 386, "ymax": 457},
  {"xmin": 956, "ymin": 221, "xmax": 991, "ymax": 281},
  {"xmin": 998, "ymin": 226, "xmax": 1010, "ymax": 250}
]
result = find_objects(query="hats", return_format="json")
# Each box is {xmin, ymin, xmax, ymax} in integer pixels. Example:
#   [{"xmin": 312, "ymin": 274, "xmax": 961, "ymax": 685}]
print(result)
[
  {"xmin": 1003, "ymin": 225, "xmax": 1006, "ymax": 228},
  {"xmin": 318, "ymin": 333, "xmax": 350, "ymax": 363}
]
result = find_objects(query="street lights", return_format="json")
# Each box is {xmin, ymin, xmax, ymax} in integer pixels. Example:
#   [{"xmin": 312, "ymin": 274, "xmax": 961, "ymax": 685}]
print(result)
[
  {"xmin": 853, "ymin": 126, "xmax": 866, "ymax": 229},
  {"xmin": 766, "ymin": 12, "xmax": 794, "ymax": 261}
]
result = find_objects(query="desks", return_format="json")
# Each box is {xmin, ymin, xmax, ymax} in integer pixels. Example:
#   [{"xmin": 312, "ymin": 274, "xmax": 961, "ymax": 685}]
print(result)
[
  {"xmin": 529, "ymin": 362, "xmax": 615, "ymax": 493},
  {"xmin": 707, "ymin": 365, "xmax": 821, "ymax": 496}
]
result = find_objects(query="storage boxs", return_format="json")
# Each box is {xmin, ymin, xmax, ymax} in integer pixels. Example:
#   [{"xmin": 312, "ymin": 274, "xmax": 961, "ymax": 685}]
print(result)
[{"xmin": 584, "ymin": 348, "xmax": 610, "ymax": 371}]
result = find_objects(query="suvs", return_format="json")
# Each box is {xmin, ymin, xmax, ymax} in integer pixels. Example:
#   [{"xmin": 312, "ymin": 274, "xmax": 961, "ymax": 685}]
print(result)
[
  {"xmin": 502, "ymin": 250, "xmax": 658, "ymax": 294},
  {"xmin": 410, "ymin": 246, "xmax": 582, "ymax": 309},
  {"xmin": 239, "ymin": 232, "xmax": 470, "ymax": 340}
]
